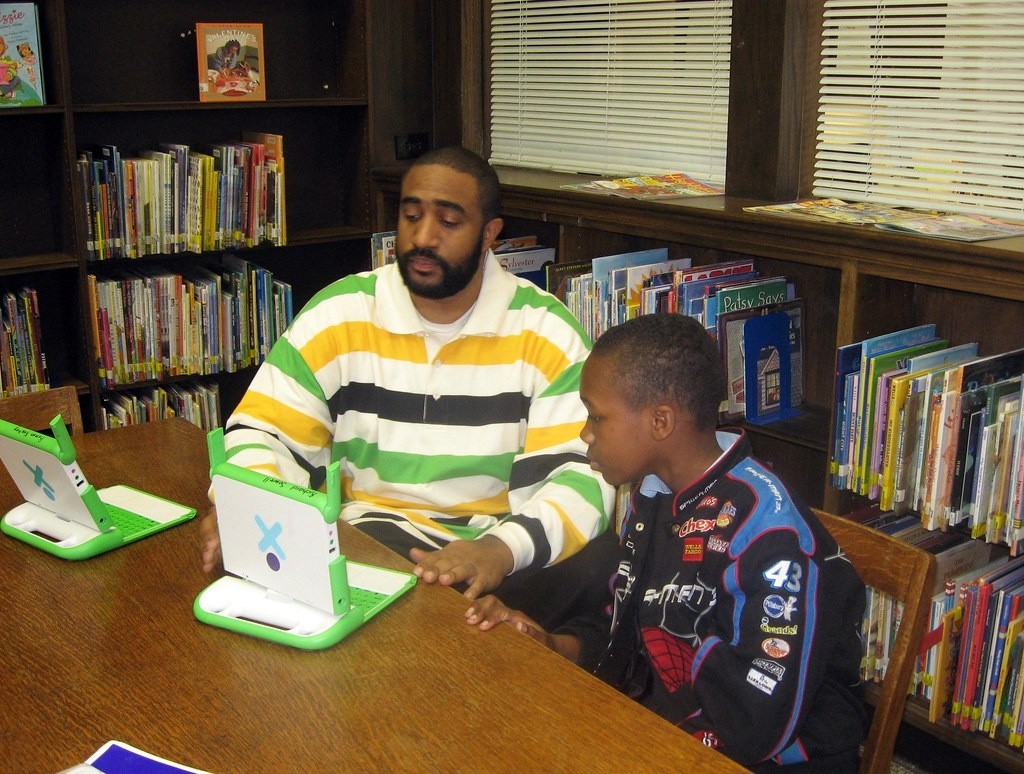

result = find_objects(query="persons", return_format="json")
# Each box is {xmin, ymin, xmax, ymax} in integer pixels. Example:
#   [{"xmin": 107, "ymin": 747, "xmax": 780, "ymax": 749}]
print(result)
[
  {"xmin": 465, "ymin": 311, "xmax": 877, "ymax": 774},
  {"xmin": 199, "ymin": 147, "xmax": 617, "ymax": 603}
]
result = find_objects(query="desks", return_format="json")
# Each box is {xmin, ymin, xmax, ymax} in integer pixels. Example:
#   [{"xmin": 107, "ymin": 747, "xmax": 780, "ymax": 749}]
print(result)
[{"xmin": 0, "ymin": 418, "xmax": 753, "ymax": 774}]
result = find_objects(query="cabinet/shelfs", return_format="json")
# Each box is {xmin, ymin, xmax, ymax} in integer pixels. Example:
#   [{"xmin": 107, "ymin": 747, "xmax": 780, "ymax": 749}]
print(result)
[
  {"xmin": 0, "ymin": 0, "xmax": 379, "ymax": 434},
  {"xmin": 377, "ymin": 155, "xmax": 1024, "ymax": 774}
]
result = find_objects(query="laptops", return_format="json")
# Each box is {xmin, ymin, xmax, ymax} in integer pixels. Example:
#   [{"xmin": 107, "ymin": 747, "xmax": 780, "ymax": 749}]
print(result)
[
  {"xmin": 193, "ymin": 426, "xmax": 417, "ymax": 653},
  {"xmin": 0, "ymin": 414, "xmax": 197, "ymax": 562}
]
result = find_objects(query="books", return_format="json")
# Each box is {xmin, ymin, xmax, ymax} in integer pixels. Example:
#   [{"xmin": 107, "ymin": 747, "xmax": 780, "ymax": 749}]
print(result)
[
  {"xmin": 742, "ymin": 197, "xmax": 1024, "ymax": 243},
  {"xmin": 545, "ymin": 247, "xmax": 807, "ymax": 428},
  {"xmin": 840, "ymin": 504, "xmax": 1024, "ymax": 751},
  {"xmin": 560, "ymin": 172, "xmax": 725, "ymax": 201},
  {"xmin": 195, "ymin": 22, "xmax": 266, "ymax": 102},
  {"xmin": 0, "ymin": 286, "xmax": 51, "ymax": 399},
  {"xmin": 827, "ymin": 321, "xmax": 1024, "ymax": 557},
  {"xmin": 370, "ymin": 230, "xmax": 556, "ymax": 293},
  {"xmin": 100, "ymin": 378, "xmax": 224, "ymax": 434},
  {"xmin": 87, "ymin": 255, "xmax": 294, "ymax": 390},
  {"xmin": 79, "ymin": 129, "xmax": 287, "ymax": 261},
  {"xmin": 0, "ymin": 2, "xmax": 47, "ymax": 108}
]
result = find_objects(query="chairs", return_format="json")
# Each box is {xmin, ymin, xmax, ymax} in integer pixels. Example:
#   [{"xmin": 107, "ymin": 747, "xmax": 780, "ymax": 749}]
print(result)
[
  {"xmin": 0, "ymin": 386, "xmax": 85, "ymax": 436},
  {"xmin": 808, "ymin": 507, "xmax": 937, "ymax": 774}
]
